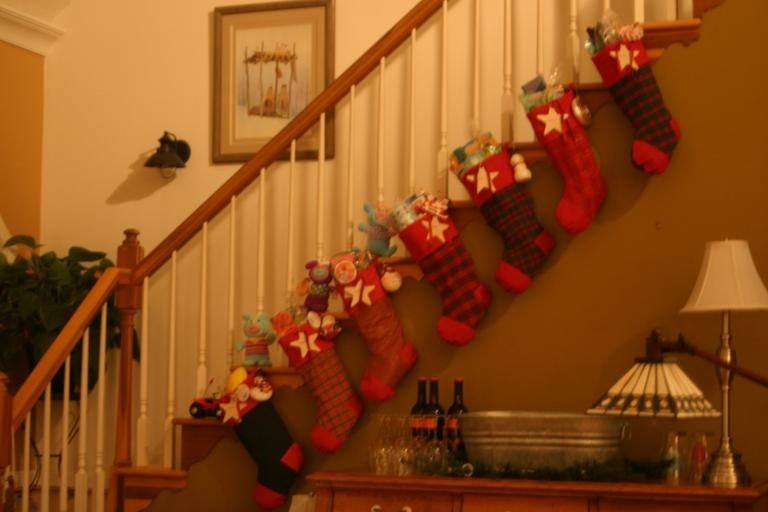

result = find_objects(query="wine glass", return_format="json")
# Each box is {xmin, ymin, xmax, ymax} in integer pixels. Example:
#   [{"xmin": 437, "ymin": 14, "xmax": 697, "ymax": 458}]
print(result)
[{"xmin": 361, "ymin": 413, "xmax": 459, "ymax": 478}]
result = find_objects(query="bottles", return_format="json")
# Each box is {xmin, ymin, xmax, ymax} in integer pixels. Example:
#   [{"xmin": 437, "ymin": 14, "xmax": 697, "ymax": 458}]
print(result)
[
  {"xmin": 410, "ymin": 376, "xmax": 467, "ymax": 466},
  {"xmin": 688, "ymin": 429, "xmax": 707, "ymax": 482},
  {"xmin": 662, "ymin": 430, "xmax": 678, "ymax": 484}
]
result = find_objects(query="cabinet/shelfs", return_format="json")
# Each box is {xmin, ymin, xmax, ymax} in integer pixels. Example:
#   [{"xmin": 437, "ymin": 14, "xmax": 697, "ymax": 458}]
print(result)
[{"xmin": 302, "ymin": 465, "xmax": 766, "ymax": 512}]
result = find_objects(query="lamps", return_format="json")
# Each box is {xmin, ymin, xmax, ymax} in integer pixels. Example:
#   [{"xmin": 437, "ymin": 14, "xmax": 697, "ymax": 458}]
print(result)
[
  {"xmin": 581, "ymin": 328, "xmax": 768, "ymax": 421},
  {"xmin": 674, "ymin": 237, "xmax": 767, "ymax": 490},
  {"xmin": 143, "ymin": 130, "xmax": 192, "ymax": 180}
]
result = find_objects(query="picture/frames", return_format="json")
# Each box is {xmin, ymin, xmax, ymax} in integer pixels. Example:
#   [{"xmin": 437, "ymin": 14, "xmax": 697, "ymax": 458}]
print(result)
[{"xmin": 211, "ymin": 0, "xmax": 337, "ymax": 163}]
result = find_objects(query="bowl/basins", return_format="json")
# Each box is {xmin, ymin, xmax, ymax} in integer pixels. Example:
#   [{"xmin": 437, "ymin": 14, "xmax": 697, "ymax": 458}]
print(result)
[{"xmin": 456, "ymin": 409, "xmax": 625, "ymax": 476}]
